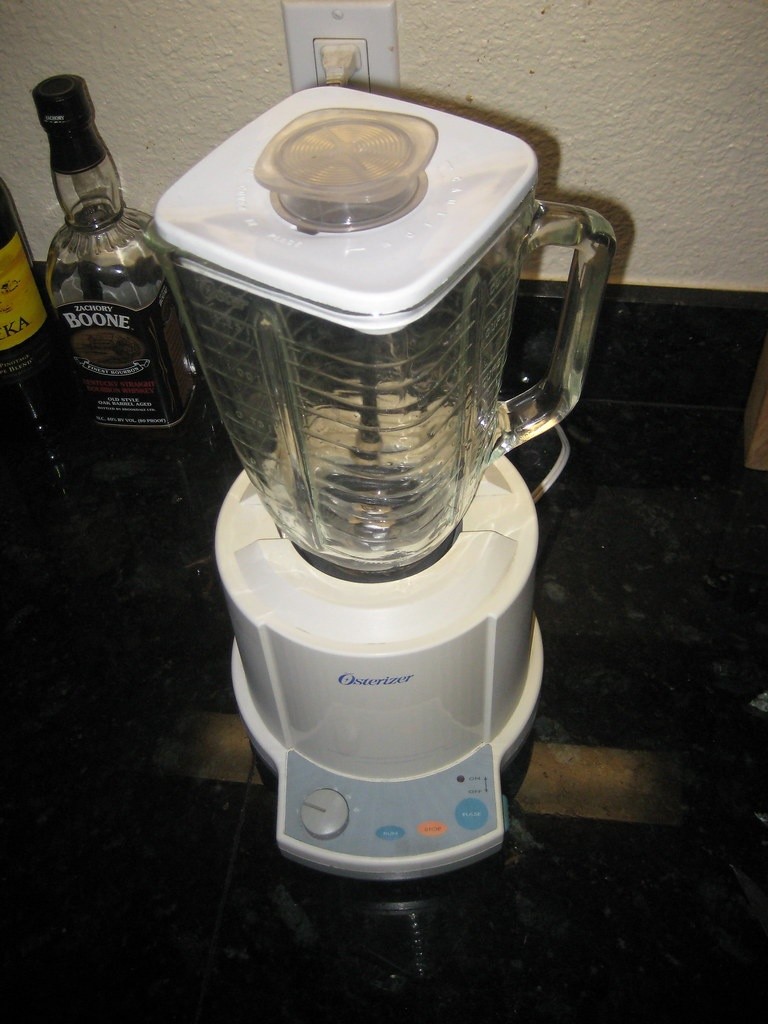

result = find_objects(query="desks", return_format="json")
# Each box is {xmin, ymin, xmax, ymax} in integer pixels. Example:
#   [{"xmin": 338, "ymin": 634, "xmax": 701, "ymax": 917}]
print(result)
[{"xmin": 0, "ymin": 367, "xmax": 767, "ymax": 1024}]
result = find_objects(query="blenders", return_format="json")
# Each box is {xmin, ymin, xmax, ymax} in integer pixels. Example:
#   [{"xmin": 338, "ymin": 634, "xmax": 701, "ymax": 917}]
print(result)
[{"xmin": 153, "ymin": 83, "xmax": 627, "ymax": 888}]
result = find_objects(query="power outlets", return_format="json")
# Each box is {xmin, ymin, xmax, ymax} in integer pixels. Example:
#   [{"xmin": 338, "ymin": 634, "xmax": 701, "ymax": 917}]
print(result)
[{"xmin": 282, "ymin": 4, "xmax": 402, "ymax": 107}]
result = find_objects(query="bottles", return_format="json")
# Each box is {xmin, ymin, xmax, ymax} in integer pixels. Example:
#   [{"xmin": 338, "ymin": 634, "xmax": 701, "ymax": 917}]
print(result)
[
  {"xmin": 32, "ymin": 74, "xmax": 198, "ymax": 430},
  {"xmin": 0, "ymin": 175, "xmax": 58, "ymax": 381}
]
